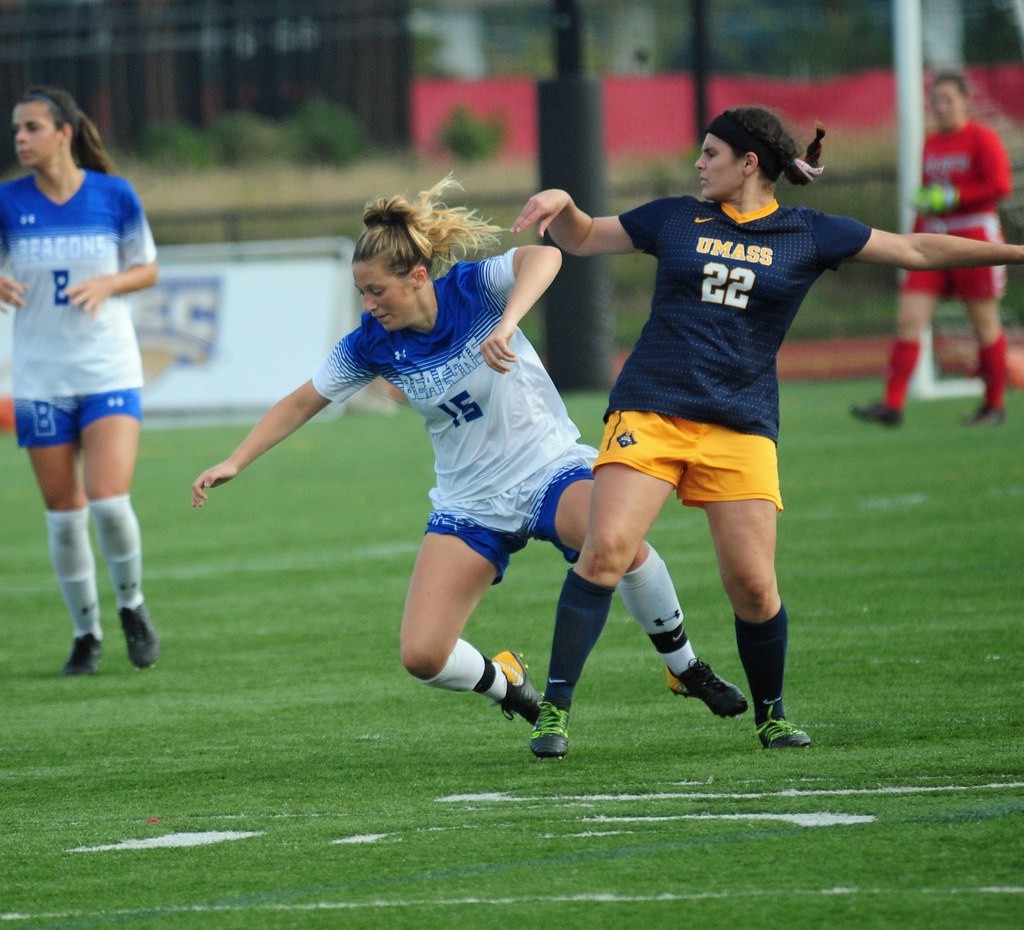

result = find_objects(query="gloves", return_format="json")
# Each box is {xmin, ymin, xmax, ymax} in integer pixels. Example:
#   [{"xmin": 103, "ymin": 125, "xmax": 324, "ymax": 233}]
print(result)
[{"xmin": 905, "ymin": 184, "xmax": 961, "ymax": 213}]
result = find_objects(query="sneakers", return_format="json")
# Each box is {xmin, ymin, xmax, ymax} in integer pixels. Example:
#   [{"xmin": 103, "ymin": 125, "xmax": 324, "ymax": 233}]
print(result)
[
  {"xmin": 530, "ymin": 698, "xmax": 569, "ymax": 759},
  {"xmin": 666, "ymin": 657, "xmax": 747, "ymax": 718},
  {"xmin": 491, "ymin": 650, "xmax": 545, "ymax": 726},
  {"xmin": 62, "ymin": 631, "xmax": 103, "ymax": 674},
  {"xmin": 117, "ymin": 597, "xmax": 160, "ymax": 667},
  {"xmin": 753, "ymin": 704, "xmax": 813, "ymax": 748}
]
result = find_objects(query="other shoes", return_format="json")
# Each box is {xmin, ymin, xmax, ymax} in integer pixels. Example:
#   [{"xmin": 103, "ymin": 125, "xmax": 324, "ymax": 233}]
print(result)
[
  {"xmin": 963, "ymin": 406, "xmax": 1004, "ymax": 428},
  {"xmin": 853, "ymin": 401, "xmax": 901, "ymax": 426}
]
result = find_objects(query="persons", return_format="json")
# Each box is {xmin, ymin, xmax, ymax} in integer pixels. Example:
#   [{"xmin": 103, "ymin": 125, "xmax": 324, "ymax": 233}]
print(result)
[
  {"xmin": 508, "ymin": 106, "xmax": 1024, "ymax": 758},
  {"xmin": 0, "ymin": 87, "xmax": 159, "ymax": 679},
  {"xmin": 190, "ymin": 170, "xmax": 747, "ymax": 726},
  {"xmin": 849, "ymin": 70, "xmax": 1011, "ymax": 429}
]
